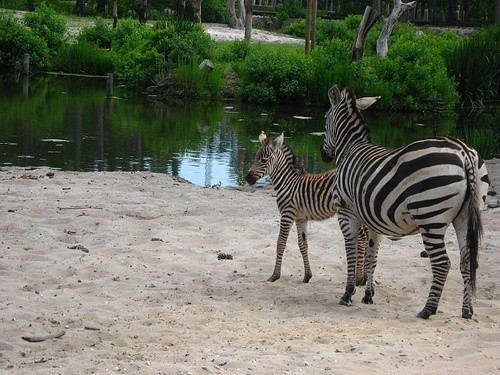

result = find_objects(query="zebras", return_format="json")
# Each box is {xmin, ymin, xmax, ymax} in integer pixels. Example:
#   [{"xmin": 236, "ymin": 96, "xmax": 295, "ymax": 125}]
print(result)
[
  {"xmin": 245, "ymin": 130, "xmax": 369, "ymax": 286},
  {"xmin": 319, "ymin": 83, "xmax": 491, "ymax": 320}
]
